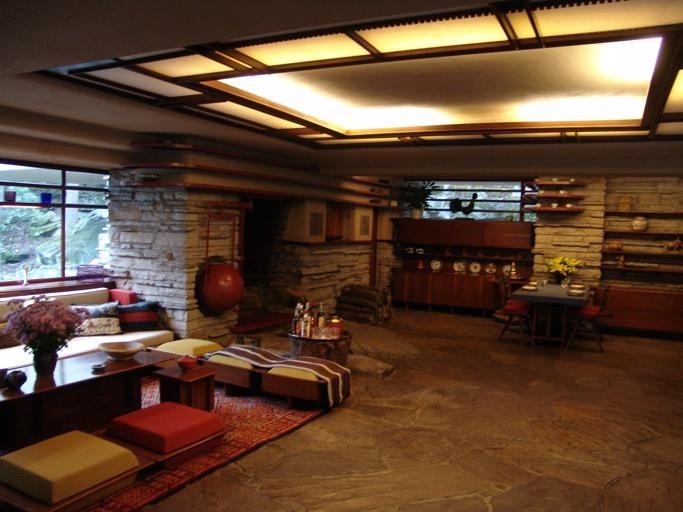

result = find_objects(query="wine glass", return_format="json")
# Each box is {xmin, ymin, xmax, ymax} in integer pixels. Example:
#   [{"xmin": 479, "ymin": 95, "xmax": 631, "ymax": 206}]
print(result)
[{"xmin": 560, "ymin": 279, "xmax": 568, "ymax": 295}]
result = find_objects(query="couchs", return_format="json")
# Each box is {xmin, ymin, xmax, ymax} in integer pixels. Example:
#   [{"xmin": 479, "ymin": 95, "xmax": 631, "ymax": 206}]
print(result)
[{"xmin": 0, "ymin": 281, "xmax": 174, "ymax": 370}]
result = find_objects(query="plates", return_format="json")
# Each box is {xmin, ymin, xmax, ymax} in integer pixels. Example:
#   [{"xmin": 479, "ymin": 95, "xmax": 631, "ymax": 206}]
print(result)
[
  {"xmin": 92, "ymin": 364, "xmax": 104, "ymax": 369},
  {"xmin": 429, "ymin": 260, "xmax": 511, "ymax": 275},
  {"xmin": 568, "ymin": 283, "xmax": 585, "ymax": 297},
  {"xmin": 522, "ymin": 279, "xmax": 549, "ymax": 291}
]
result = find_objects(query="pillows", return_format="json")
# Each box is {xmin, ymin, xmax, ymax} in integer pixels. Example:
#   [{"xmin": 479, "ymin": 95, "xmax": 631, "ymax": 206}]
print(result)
[
  {"xmin": 117, "ymin": 300, "xmax": 160, "ymax": 330},
  {"xmin": 68, "ymin": 301, "xmax": 122, "ymax": 336}
]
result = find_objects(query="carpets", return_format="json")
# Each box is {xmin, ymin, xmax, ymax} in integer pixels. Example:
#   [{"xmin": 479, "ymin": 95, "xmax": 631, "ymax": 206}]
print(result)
[{"xmin": 0, "ymin": 375, "xmax": 327, "ymax": 512}]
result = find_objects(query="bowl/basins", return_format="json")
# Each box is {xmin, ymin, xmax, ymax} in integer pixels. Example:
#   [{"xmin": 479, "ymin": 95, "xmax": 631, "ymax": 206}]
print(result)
[
  {"xmin": 606, "ymin": 239, "xmax": 623, "ymax": 251},
  {"xmin": 98, "ymin": 341, "xmax": 144, "ymax": 361},
  {"xmin": 175, "ymin": 356, "xmax": 196, "ymax": 369}
]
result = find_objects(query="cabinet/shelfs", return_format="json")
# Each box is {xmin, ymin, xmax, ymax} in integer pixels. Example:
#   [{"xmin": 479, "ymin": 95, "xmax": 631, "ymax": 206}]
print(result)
[
  {"xmin": 523, "ymin": 181, "xmax": 585, "ymax": 211},
  {"xmin": 390, "ymin": 217, "xmax": 532, "ymax": 312},
  {"xmin": 601, "ymin": 210, "xmax": 683, "ymax": 332}
]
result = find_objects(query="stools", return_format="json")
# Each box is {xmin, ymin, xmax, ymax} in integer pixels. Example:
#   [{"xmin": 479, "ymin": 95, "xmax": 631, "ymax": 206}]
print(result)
[
  {"xmin": 1, "ymin": 430, "xmax": 156, "ymax": 512},
  {"xmin": 261, "ymin": 357, "xmax": 351, "ymax": 410},
  {"xmin": 207, "ymin": 345, "xmax": 265, "ymax": 394},
  {"xmin": 106, "ymin": 401, "xmax": 235, "ymax": 469},
  {"xmin": 157, "ymin": 339, "xmax": 224, "ymax": 357}
]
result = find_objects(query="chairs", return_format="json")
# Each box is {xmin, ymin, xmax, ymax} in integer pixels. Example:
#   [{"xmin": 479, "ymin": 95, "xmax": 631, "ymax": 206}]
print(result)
[
  {"xmin": 498, "ymin": 278, "xmax": 530, "ymax": 344},
  {"xmin": 564, "ymin": 285, "xmax": 609, "ymax": 352}
]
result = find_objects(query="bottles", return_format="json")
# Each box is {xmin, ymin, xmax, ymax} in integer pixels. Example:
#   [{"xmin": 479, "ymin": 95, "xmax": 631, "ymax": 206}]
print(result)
[
  {"xmin": 631, "ymin": 216, "xmax": 647, "ymax": 231},
  {"xmin": 509, "ymin": 260, "xmax": 518, "ymax": 278},
  {"xmin": 292, "ymin": 301, "xmax": 347, "ymax": 339}
]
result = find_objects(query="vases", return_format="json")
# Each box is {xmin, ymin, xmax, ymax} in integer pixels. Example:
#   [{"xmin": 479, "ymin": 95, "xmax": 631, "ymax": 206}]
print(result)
[
  {"xmin": 555, "ymin": 273, "xmax": 565, "ymax": 284},
  {"xmin": 34, "ymin": 350, "xmax": 58, "ymax": 376}
]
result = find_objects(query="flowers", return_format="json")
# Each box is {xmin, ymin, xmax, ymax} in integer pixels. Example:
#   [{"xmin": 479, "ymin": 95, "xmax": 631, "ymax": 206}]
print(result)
[
  {"xmin": 3, "ymin": 295, "xmax": 92, "ymax": 353},
  {"xmin": 544, "ymin": 257, "xmax": 582, "ymax": 277}
]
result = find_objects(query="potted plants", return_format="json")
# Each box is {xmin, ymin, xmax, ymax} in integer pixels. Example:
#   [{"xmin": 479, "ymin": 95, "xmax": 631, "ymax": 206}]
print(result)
[{"xmin": 397, "ymin": 179, "xmax": 440, "ymax": 219}]
